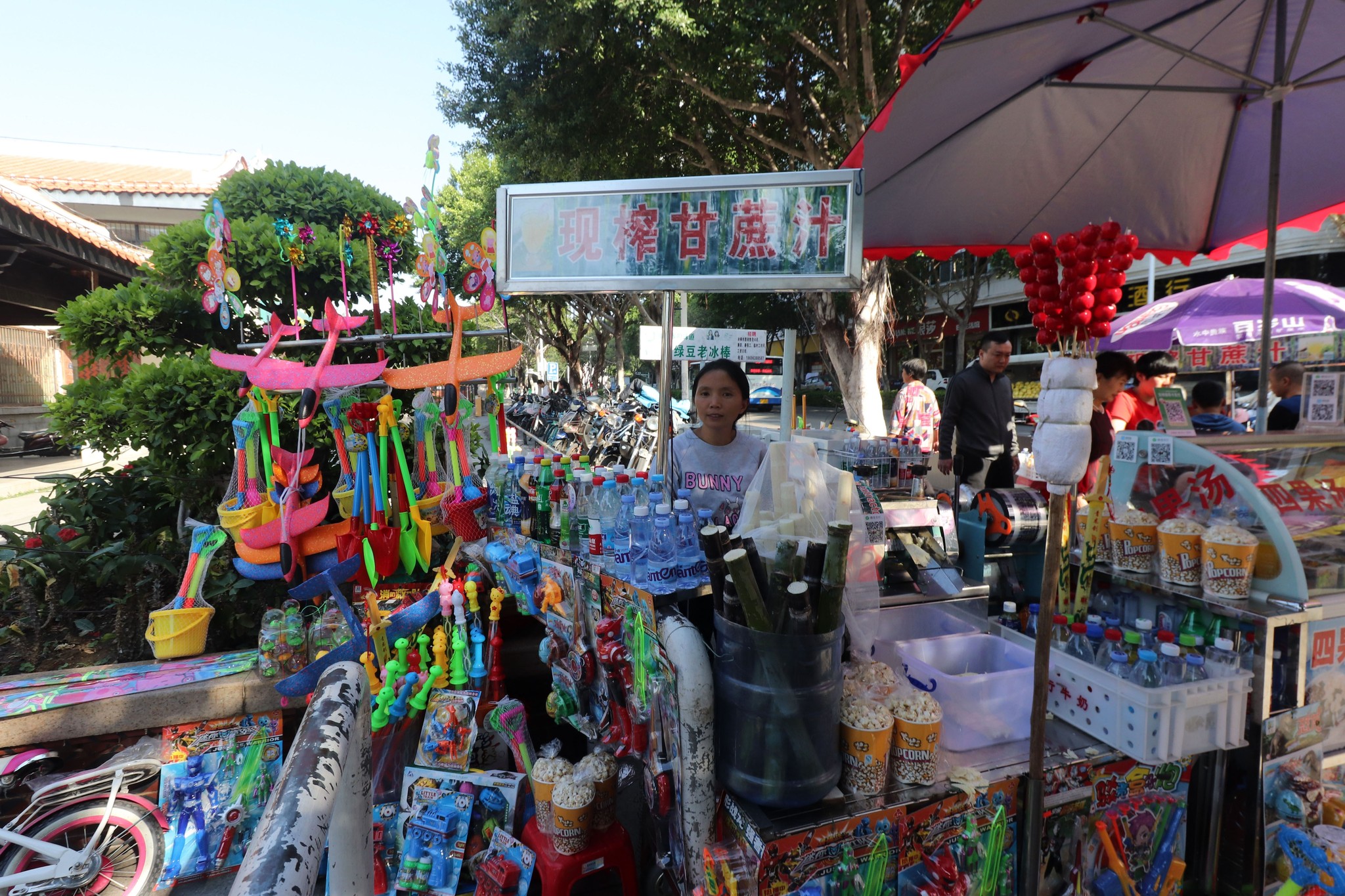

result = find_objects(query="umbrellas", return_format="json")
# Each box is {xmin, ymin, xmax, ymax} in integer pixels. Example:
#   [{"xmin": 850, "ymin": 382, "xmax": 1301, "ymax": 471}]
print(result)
[
  {"xmin": 836, "ymin": 1, "xmax": 1345, "ymax": 433},
  {"xmin": 1110, "ymin": 273, "xmax": 1345, "ymax": 421}
]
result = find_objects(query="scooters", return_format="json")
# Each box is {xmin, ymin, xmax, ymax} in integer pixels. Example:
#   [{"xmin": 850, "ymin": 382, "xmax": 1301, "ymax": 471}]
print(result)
[
  {"xmin": 0, "ymin": 419, "xmax": 74, "ymax": 458},
  {"xmin": 505, "ymin": 378, "xmax": 691, "ymax": 478}
]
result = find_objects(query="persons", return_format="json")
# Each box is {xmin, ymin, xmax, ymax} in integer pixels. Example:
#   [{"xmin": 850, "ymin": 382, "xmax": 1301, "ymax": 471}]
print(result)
[
  {"xmin": 887, "ymin": 358, "xmax": 942, "ymax": 456},
  {"xmin": 938, "ymin": 332, "xmax": 1020, "ymax": 497},
  {"xmin": 645, "ymin": 358, "xmax": 767, "ymax": 527},
  {"xmin": 1074, "ymin": 351, "xmax": 1309, "ymax": 511},
  {"xmin": 537, "ymin": 379, "xmax": 572, "ymax": 400}
]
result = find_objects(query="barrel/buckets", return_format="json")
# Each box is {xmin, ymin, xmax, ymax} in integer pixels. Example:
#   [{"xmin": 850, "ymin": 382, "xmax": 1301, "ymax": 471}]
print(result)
[
  {"xmin": 218, "ymin": 493, "xmax": 270, "ymax": 542},
  {"xmin": 332, "ymin": 475, "xmax": 364, "ymax": 519},
  {"xmin": 439, "ymin": 487, "xmax": 488, "ymax": 542},
  {"xmin": 145, "ymin": 608, "xmax": 215, "ymax": 658},
  {"xmin": 413, "ymin": 482, "xmax": 455, "ymax": 536},
  {"xmin": 712, "ymin": 608, "xmax": 845, "ymax": 809}
]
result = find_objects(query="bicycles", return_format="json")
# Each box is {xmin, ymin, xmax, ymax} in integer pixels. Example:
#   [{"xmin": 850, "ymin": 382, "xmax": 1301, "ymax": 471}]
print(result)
[
  {"xmin": 819, "ymin": 395, "xmax": 859, "ymax": 433},
  {"xmin": 0, "ymin": 747, "xmax": 170, "ymax": 896}
]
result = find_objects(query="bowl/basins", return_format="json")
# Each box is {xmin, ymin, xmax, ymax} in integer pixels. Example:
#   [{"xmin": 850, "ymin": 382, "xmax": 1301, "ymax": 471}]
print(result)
[{"xmin": 1302, "ymin": 558, "xmax": 1342, "ymax": 590}]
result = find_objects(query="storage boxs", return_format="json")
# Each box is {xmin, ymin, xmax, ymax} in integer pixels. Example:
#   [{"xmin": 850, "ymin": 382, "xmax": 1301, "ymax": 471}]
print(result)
[
  {"xmin": 849, "ymin": 596, "xmax": 1256, "ymax": 765},
  {"xmin": 1296, "ymin": 536, "xmax": 1345, "ymax": 588}
]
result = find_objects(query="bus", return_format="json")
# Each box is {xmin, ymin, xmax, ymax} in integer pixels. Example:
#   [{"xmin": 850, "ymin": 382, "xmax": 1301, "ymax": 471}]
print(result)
[{"xmin": 689, "ymin": 354, "xmax": 783, "ymax": 412}]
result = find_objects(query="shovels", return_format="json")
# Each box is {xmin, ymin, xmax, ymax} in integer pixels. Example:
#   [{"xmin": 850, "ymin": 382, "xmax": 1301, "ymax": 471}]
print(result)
[
  {"xmin": 224, "ymin": 411, "xmax": 263, "ymax": 512},
  {"xmin": 413, "ymin": 403, "xmax": 445, "ymax": 517},
  {"xmin": 438, "ymin": 398, "xmax": 483, "ymax": 507},
  {"xmin": 324, "ymin": 395, "xmax": 433, "ymax": 588}
]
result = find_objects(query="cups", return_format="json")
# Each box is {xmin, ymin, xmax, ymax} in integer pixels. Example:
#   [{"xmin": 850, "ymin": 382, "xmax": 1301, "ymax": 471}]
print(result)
[
  {"xmin": 1156, "ymin": 525, "xmax": 1203, "ymax": 586},
  {"xmin": 1200, "ymin": 535, "xmax": 1260, "ymax": 599},
  {"xmin": 1108, "ymin": 519, "xmax": 1156, "ymax": 572},
  {"xmin": 1075, "ymin": 512, "xmax": 1110, "ymax": 562},
  {"xmin": 841, "ymin": 684, "xmax": 942, "ymax": 795},
  {"xmin": 530, "ymin": 762, "xmax": 619, "ymax": 855}
]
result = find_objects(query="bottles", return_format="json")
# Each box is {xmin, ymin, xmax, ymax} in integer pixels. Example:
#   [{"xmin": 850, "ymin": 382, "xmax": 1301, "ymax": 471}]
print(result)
[
  {"xmin": 1050, "ymin": 614, "xmax": 1074, "ymax": 653},
  {"xmin": 1063, "ymin": 623, "xmax": 1094, "ymax": 665},
  {"xmin": 307, "ymin": 608, "xmax": 356, "ymax": 666},
  {"xmin": 1151, "ymin": 629, "xmax": 1182, "ymax": 689},
  {"xmin": 1084, "ymin": 614, "xmax": 1103, "ymax": 654},
  {"xmin": 1095, "ymin": 616, "xmax": 1162, "ymax": 689},
  {"xmin": 1203, "ymin": 637, "xmax": 1239, "ymax": 679},
  {"xmin": 1018, "ymin": 448, "xmax": 1035, "ymax": 477},
  {"xmin": 1178, "ymin": 633, "xmax": 1208, "ymax": 683},
  {"xmin": 841, "ymin": 431, "xmax": 923, "ymax": 488},
  {"xmin": 485, "ymin": 452, "xmax": 526, "ymax": 534},
  {"xmin": 521, "ymin": 455, "xmax": 626, "ymax": 568},
  {"xmin": 600, "ymin": 471, "xmax": 715, "ymax": 595},
  {"xmin": 997, "ymin": 601, "xmax": 1022, "ymax": 633},
  {"xmin": 257, "ymin": 616, "xmax": 307, "ymax": 680},
  {"xmin": 1024, "ymin": 604, "xmax": 1040, "ymax": 640}
]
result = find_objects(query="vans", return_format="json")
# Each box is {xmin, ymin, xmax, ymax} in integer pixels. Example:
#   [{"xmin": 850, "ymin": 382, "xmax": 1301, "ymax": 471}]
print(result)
[{"xmin": 965, "ymin": 350, "xmax": 1080, "ymax": 425}]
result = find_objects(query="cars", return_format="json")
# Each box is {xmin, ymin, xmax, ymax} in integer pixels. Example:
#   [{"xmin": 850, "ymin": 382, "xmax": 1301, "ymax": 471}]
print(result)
[
  {"xmin": 879, "ymin": 374, "xmax": 902, "ymax": 391},
  {"xmin": 794, "ymin": 375, "xmax": 840, "ymax": 392}
]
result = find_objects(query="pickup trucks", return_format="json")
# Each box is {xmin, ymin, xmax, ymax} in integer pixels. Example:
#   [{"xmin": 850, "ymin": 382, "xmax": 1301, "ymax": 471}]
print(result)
[{"xmin": 900, "ymin": 369, "xmax": 951, "ymax": 392}]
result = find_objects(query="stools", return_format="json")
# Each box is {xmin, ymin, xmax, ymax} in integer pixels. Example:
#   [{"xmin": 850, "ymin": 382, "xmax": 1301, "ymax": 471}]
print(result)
[{"xmin": 520, "ymin": 814, "xmax": 639, "ymax": 896}]
